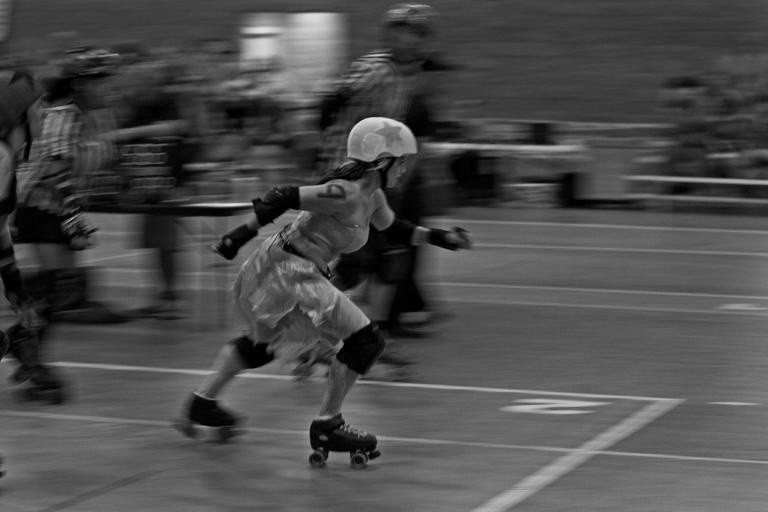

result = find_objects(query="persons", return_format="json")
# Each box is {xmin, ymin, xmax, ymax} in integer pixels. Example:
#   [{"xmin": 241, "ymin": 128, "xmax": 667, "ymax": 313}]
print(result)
[
  {"xmin": 1, "ymin": 41, "xmax": 309, "ymax": 404},
  {"xmin": 288, "ymin": 6, "xmax": 474, "ymax": 381},
  {"xmin": 654, "ymin": 71, "xmax": 768, "ymax": 195},
  {"xmin": 179, "ymin": 116, "xmax": 469, "ymax": 455}
]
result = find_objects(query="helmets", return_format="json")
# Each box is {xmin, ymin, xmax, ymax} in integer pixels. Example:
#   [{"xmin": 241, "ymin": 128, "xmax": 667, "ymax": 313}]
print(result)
[
  {"xmin": 346, "ymin": 115, "xmax": 419, "ymax": 163},
  {"xmin": 62, "ymin": 45, "xmax": 120, "ymax": 80},
  {"xmin": 381, "ymin": 3, "xmax": 439, "ymax": 34}
]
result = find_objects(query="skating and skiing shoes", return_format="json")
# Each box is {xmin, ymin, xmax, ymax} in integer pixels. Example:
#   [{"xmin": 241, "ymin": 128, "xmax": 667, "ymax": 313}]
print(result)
[
  {"xmin": 12, "ymin": 359, "xmax": 60, "ymax": 395},
  {"xmin": 309, "ymin": 413, "xmax": 377, "ymax": 454},
  {"xmin": 183, "ymin": 388, "xmax": 237, "ymax": 427},
  {"xmin": 303, "ymin": 335, "xmax": 415, "ymax": 367}
]
